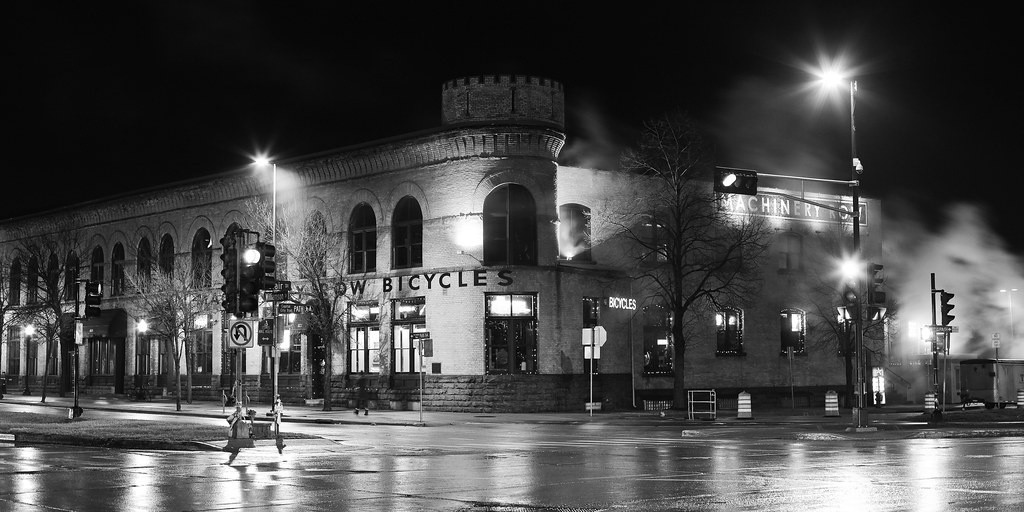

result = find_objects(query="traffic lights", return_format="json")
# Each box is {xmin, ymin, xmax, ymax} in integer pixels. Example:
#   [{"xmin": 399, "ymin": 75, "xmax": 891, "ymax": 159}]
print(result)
[
  {"xmin": 842, "ymin": 260, "xmax": 860, "ymax": 307},
  {"xmin": 715, "ymin": 166, "xmax": 759, "ymax": 195},
  {"xmin": 867, "ymin": 262, "xmax": 887, "ymax": 320},
  {"xmin": 86, "ymin": 279, "xmax": 102, "ymax": 318},
  {"xmin": 220, "ymin": 233, "xmax": 238, "ymax": 313},
  {"xmin": 257, "ymin": 242, "xmax": 277, "ymax": 287},
  {"xmin": 241, "ymin": 247, "xmax": 261, "ymax": 313},
  {"xmin": 941, "ymin": 289, "xmax": 956, "ymax": 326}
]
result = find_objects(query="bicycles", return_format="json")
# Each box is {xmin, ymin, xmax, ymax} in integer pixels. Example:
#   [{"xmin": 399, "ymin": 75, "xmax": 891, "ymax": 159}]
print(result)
[{"xmin": 127, "ymin": 382, "xmax": 152, "ymax": 403}]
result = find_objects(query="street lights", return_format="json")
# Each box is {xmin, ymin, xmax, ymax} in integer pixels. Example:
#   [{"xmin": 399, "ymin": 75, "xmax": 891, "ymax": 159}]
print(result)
[
  {"xmin": 23, "ymin": 325, "xmax": 34, "ymax": 395},
  {"xmin": 1000, "ymin": 288, "xmax": 1019, "ymax": 337},
  {"xmin": 820, "ymin": 72, "xmax": 873, "ymax": 435},
  {"xmin": 138, "ymin": 319, "xmax": 148, "ymax": 400}
]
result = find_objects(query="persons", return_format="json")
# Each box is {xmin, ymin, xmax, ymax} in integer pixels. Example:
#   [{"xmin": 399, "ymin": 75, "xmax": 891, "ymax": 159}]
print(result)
[
  {"xmin": 0, "ymin": 371, "xmax": 7, "ymax": 394},
  {"xmin": 229, "ymin": 381, "xmax": 237, "ymax": 399},
  {"xmin": 226, "ymin": 406, "xmax": 246, "ymax": 431},
  {"xmin": 341, "ymin": 374, "xmax": 353, "ymax": 409},
  {"xmin": 352, "ymin": 370, "xmax": 370, "ymax": 416}
]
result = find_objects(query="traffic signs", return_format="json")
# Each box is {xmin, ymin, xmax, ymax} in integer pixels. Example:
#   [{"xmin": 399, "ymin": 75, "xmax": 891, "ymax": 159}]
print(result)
[
  {"xmin": 275, "ymin": 281, "xmax": 294, "ymax": 291},
  {"xmin": 411, "ymin": 332, "xmax": 431, "ymax": 338},
  {"xmin": 280, "ymin": 303, "xmax": 315, "ymax": 314},
  {"xmin": 259, "ymin": 319, "xmax": 275, "ymax": 346},
  {"xmin": 930, "ymin": 325, "xmax": 959, "ymax": 333},
  {"xmin": 265, "ymin": 292, "xmax": 291, "ymax": 302}
]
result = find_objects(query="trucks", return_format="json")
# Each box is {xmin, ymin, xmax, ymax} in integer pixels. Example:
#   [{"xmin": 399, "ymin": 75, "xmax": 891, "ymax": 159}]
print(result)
[{"xmin": 960, "ymin": 359, "xmax": 1024, "ymax": 409}]
